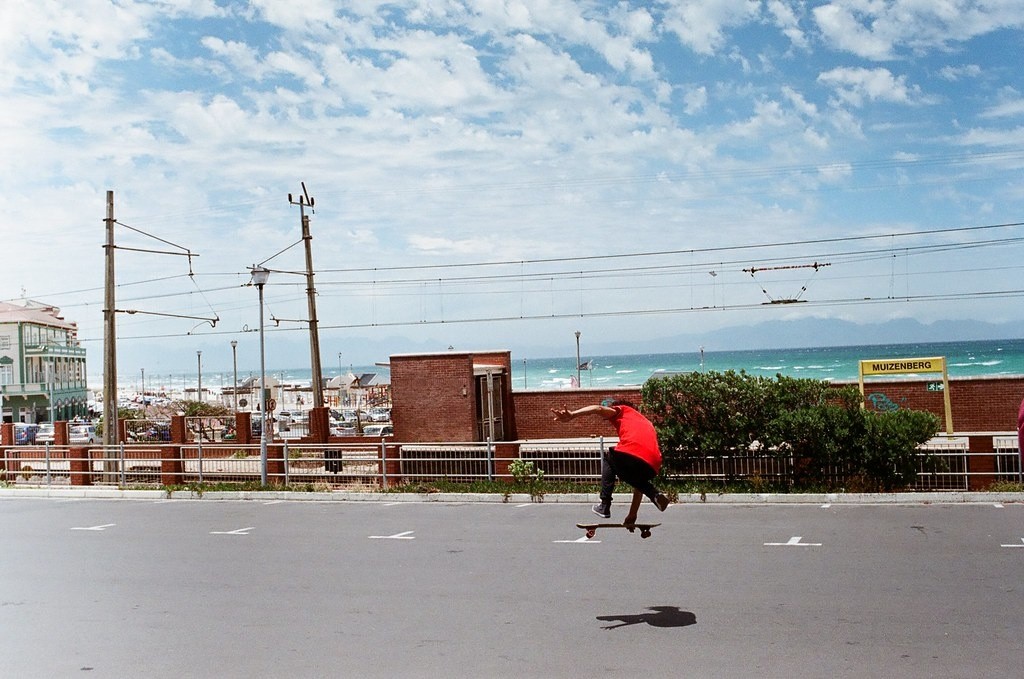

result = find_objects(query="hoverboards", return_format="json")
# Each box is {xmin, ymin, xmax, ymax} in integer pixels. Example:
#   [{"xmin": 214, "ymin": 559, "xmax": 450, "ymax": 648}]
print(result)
[{"xmin": 576, "ymin": 522, "xmax": 661, "ymax": 539}]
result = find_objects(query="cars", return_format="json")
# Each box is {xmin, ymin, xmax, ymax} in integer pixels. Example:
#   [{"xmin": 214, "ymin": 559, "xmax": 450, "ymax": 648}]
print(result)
[
  {"xmin": 69, "ymin": 426, "xmax": 103, "ymax": 444},
  {"xmin": 15, "ymin": 423, "xmax": 40, "ymax": 445},
  {"xmin": 221, "ymin": 406, "xmax": 393, "ymax": 438},
  {"xmin": 35, "ymin": 424, "xmax": 56, "ymax": 445},
  {"xmin": 96, "ymin": 391, "xmax": 185, "ymax": 411},
  {"xmin": 127, "ymin": 422, "xmax": 170, "ymax": 441}
]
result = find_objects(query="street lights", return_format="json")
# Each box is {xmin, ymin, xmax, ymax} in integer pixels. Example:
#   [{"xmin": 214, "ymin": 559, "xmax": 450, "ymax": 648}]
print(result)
[
  {"xmin": 231, "ymin": 340, "xmax": 238, "ymax": 416},
  {"xmin": 575, "ymin": 330, "xmax": 582, "ymax": 388},
  {"xmin": 251, "ymin": 266, "xmax": 271, "ymax": 486},
  {"xmin": 140, "ymin": 368, "xmax": 145, "ymax": 413},
  {"xmin": 196, "ymin": 350, "xmax": 202, "ymax": 402}
]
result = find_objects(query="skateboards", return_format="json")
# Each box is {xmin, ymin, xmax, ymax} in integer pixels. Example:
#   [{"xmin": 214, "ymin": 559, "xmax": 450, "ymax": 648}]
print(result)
[{"xmin": 575, "ymin": 522, "xmax": 663, "ymax": 539}]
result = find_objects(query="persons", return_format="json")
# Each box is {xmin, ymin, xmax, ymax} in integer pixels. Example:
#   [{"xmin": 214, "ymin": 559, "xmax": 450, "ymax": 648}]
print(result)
[{"xmin": 550, "ymin": 399, "xmax": 671, "ymax": 523}]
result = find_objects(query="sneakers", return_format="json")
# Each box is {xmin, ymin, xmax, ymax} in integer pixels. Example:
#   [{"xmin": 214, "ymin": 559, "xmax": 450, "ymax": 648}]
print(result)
[
  {"xmin": 592, "ymin": 502, "xmax": 611, "ymax": 519},
  {"xmin": 653, "ymin": 493, "xmax": 670, "ymax": 512}
]
